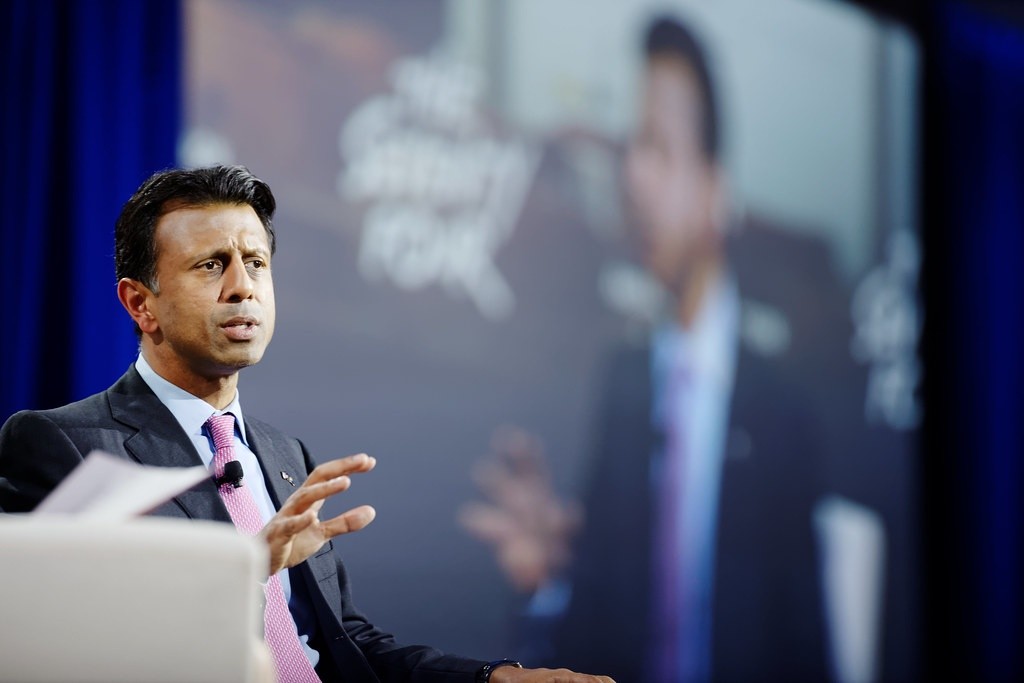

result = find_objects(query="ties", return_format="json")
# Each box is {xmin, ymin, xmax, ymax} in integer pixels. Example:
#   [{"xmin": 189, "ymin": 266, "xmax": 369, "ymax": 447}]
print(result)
[
  {"xmin": 649, "ymin": 361, "xmax": 688, "ymax": 681},
  {"xmin": 205, "ymin": 413, "xmax": 322, "ymax": 683}
]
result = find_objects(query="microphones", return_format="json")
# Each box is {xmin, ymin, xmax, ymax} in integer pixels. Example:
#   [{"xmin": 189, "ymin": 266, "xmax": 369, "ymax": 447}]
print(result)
[{"xmin": 224, "ymin": 460, "xmax": 244, "ymax": 489}]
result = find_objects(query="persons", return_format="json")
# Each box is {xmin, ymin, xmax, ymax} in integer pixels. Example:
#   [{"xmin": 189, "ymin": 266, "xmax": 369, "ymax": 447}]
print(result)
[
  {"xmin": 0, "ymin": 164, "xmax": 614, "ymax": 683},
  {"xmin": 461, "ymin": 17, "xmax": 920, "ymax": 683}
]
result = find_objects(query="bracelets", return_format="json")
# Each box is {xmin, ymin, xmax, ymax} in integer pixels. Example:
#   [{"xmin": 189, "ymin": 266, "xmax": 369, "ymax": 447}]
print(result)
[{"xmin": 478, "ymin": 656, "xmax": 530, "ymax": 683}]
